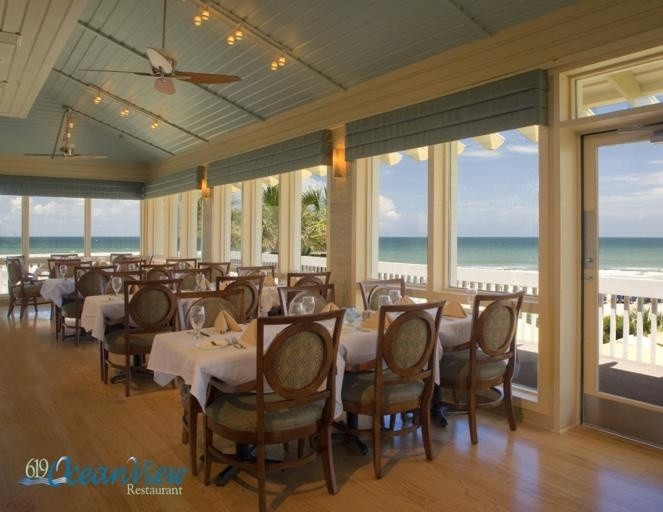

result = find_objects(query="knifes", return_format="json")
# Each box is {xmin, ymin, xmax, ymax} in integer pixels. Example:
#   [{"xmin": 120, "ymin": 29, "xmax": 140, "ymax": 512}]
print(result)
[{"xmin": 226, "ymin": 339, "xmax": 241, "ymax": 349}]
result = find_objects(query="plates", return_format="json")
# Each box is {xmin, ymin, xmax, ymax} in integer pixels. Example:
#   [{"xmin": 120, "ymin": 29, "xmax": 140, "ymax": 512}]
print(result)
[{"xmin": 197, "ymin": 339, "xmax": 229, "ymax": 350}]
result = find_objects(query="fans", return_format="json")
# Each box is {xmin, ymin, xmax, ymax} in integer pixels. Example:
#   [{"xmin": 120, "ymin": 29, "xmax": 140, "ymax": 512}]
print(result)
[
  {"xmin": 22, "ymin": 106, "xmax": 110, "ymax": 163},
  {"xmin": 76, "ymin": 0, "xmax": 242, "ymax": 96}
]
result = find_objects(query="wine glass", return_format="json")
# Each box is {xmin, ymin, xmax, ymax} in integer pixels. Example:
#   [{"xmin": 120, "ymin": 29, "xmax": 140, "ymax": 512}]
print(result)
[
  {"xmin": 378, "ymin": 294, "xmax": 390, "ymax": 309},
  {"xmin": 197, "ymin": 274, "xmax": 205, "ymax": 291},
  {"xmin": 289, "ymin": 302, "xmax": 303, "ymax": 317},
  {"xmin": 60, "ymin": 265, "xmax": 67, "ymax": 280},
  {"xmin": 160, "ymin": 277, "xmax": 168, "ymax": 286},
  {"xmin": 190, "ymin": 306, "xmax": 205, "ymax": 341},
  {"xmin": 129, "ymin": 263, "xmax": 136, "ymax": 272},
  {"xmin": 302, "ymin": 296, "xmax": 315, "ymax": 315},
  {"xmin": 112, "ymin": 277, "xmax": 122, "ymax": 298},
  {"xmin": 390, "ymin": 289, "xmax": 401, "ymax": 304}
]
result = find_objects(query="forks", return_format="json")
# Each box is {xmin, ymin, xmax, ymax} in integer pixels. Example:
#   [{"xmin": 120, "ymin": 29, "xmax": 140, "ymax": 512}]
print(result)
[{"xmin": 231, "ymin": 336, "xmax": 247, "ymax": 349}]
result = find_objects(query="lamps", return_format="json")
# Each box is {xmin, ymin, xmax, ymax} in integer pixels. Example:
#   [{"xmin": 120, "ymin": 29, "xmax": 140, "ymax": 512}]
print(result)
[{"xmin": 155, "ymin": 78, "xmax": 176, "ymax": 96}]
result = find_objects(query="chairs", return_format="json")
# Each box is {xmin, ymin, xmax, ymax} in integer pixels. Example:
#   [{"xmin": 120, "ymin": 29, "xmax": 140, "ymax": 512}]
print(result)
[
  {"xmin": 427, "ymin": 290, "xmax": 526, "ymax": 445},
  {"xmin": 173, "ymin": 288, "xmax": 246, "ymax": 446},
  {"xmin": 359, "ymin": 276, "xmax": 408, "ymax": 311},
  {"xmin": 275, "ymin": 282, "xmax": 335, "ymax": 320},
  {"xmin": 204, "ymin": 305, "xmax": 347, "ymax": 512},
  {"xmin": 342, "ymin": 298, "xmax": 449, "ymax": 480}
]
